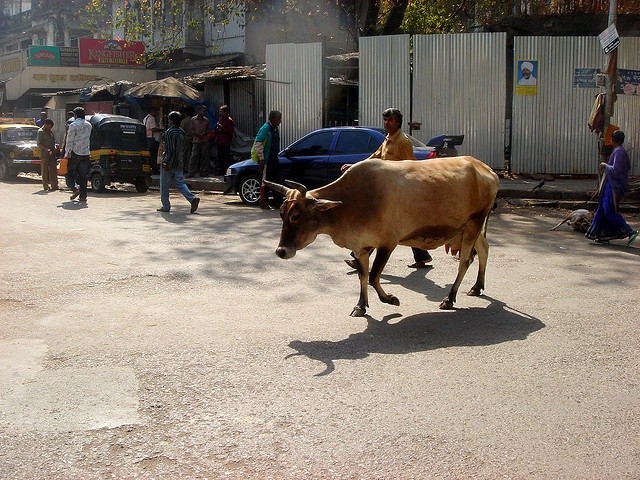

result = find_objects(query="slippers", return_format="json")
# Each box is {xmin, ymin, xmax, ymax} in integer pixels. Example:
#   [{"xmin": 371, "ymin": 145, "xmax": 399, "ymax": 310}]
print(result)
[
  {"xmin": 626, "ymin": 231, "xmax": 639, "ymax": 247},
  {"xmin": 594, "ymin": 239, "xmax": 610, "ymax": 244}
]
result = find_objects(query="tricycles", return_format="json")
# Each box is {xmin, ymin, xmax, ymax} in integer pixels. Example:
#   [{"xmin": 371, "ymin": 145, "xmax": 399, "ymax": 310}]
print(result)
[{"xmin": 85, "ymin": 115, "xmax": 151, "ymax": 192}]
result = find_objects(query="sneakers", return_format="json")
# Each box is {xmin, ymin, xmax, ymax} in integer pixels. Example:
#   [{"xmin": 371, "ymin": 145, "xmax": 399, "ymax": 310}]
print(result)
[{"xmin": 263, "ymin": 206, "xmax": 274, "ymax": 211}]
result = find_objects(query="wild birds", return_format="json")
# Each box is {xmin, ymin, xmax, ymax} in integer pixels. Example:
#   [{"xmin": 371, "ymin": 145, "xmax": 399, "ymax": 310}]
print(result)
[{"xmin": 531, "ymin": 178, "xmax": 546, "ymax": 193}]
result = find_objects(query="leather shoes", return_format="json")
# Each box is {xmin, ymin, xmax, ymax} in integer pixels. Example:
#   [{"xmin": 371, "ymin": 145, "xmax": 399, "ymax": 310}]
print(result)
[
  {"xmin": 190, "ymin": 198, "xmax": 200, "ymax": 213},
  {"xmin": 156, "ymin": 207, "xmax": 169, "ymax": 212},
  {"xmin": 69, "ymin": 189, "xmax": 81, "ymax": 199}
]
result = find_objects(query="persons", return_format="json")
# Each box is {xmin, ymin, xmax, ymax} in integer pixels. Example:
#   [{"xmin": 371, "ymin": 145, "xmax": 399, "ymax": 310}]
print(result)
[
  {"xmin": 36, "ymin": 111, "xmax": 47, "ymax": 127},
  {"xmin": 157, "ymin": 111, "xmax": 200, "ymax": 214},
  {"xmin": 213, "ymin": 105, "xmax": 235, "ymax": 177},
  {"xmin": 185, "ymin": 105, "xmax": 211, "ymax": 179},
  {"xmin": 179, "ymin": 108, "xmax": 192, "ymax": 174},
  {"xmin": 250, "ymin": 110, "xmax": 284, "ymax": 211},
  {"xmin": 66, "ymin": 111, "xmax": 74, "ymax": 120},
  {"xmin": 585, "ymin": 130, "xmax": 639, "ymax": 246},
  {"xmin": 36, "ymin": 117, "xmax": 64, "ymax": 192},
  {"xmin": 63, "ymin": 106, "xmax": 92, "ymax": 204},
  {"xmin": 142, "ymin": 107, "xmax": 165, "ymax": 176},
  {"xmin": 518, "ymin": 62, "xmax": 536, "ymax": 85},
  {"xmin": 340, "ymin": 108, "xmax": 433, "ymax": 268}
]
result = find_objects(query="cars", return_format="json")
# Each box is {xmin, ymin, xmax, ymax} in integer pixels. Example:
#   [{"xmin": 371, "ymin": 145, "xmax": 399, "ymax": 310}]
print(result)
[
  {"xmin": 224, "ymin": 126, "xmax": 464, "ymax": 205},
  {"xmin": 0, "ymin": 123, "xmax": 59, "ymax": 181}
]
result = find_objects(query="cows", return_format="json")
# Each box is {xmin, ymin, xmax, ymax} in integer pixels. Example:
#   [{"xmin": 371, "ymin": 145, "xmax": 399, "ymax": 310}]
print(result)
[{"xmin": 261, "ymin": 156, "xmax": 501, "ymax": 318}]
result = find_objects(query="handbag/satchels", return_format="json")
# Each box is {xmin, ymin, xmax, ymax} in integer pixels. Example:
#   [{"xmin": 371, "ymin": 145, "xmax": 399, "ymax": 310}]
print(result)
[{"xmin": 56, "ymin": 158, "xmax": 68, "ymax": 175}]
